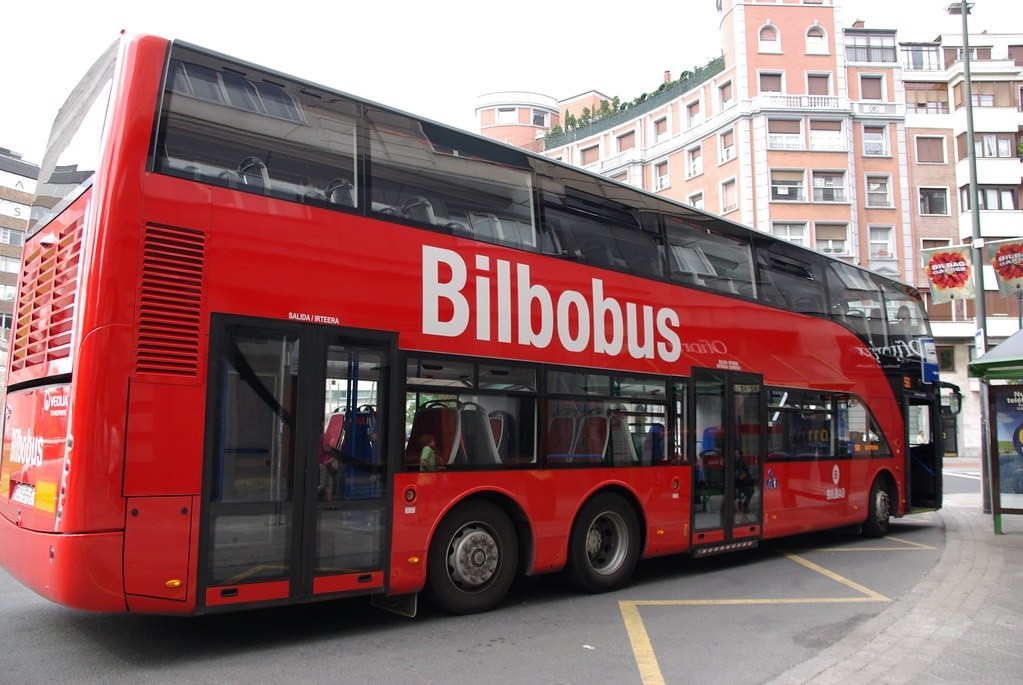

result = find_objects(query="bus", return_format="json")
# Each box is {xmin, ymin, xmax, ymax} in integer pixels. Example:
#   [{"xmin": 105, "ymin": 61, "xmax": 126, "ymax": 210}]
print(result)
[{"xmin": 0, "ymin": 28, "xmax": 964, "ymax": 621}]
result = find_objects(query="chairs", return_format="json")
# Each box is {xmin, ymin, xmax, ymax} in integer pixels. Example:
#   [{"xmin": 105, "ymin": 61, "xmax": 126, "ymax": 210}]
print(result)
[
  {"xmin": 237, "ymin": 155, "xmax": 272, "ymax": 189},
  {"xmin": 541, "ymin": 223, "xmax": 562, "ymax": 254},
  {"xmin": 889, "ymin": 320, "xmax": 908, "ymax": 345},
  {"xmin": 400, "ymin": 194, "xmax": 437, "ymax": 225},
  {"xmin": 468, "ymin": 212, "xmax": 505, "ymax": 240},
  {"xmin": 672, "ymin": 256, "xmax": 700, "ymax": 285},
  {"xmin": 324, "ymin": 176, "xmax": 355, "ymax": 206},
  {"xmin": 698, "ymin": 426, "xmax": 720, "ymax": 482},
  {"xmin": 867, "ymin": 315, "xmax": 884, "ymax": 346},
  {"xmin": 581, "ymin": 241, "xmax": 615, "ymax": 265},
  {"xmin": 740, "ymin": 281, "xmax": 777, "ymax": 303},
  {"xmin": 844, "ymin": 308, "xmax": 872, "ymax": 343},
  {"xmin": 626, "ymin": 254, "xmax": 658, "ymax": 275},
  {"xmin": 319, "ymin": 397, "xmax": 665, "ymax": 466},
  {"xmin": 704, "ymin": 274, "xmax": 736, "ymax": 293},
  {"xmin": 501, "ymin": 218, "xmax": 526, "ymax": 248},
  {"xmin": 699, "ymin": 449, "xmax": 723, "ymax": 485}
]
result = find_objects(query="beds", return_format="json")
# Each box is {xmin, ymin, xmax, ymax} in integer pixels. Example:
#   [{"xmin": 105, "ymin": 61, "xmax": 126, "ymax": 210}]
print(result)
[{"xmin": 797, "ymin": 293, "xmax": 824, "ymax": 313}]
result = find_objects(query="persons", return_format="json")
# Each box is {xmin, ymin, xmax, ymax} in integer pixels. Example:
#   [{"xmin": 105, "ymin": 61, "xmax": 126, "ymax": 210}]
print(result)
[
  {"xmin": 895, "ymin": 306, "xmax": 921, "ymax": 336},
  {"xmin": 316, "ymin": 424, "xmax": 340, "ymax": 510},
  {"xmin": 417, "ymin": 434, "xmax": 445, "ymax": 472},
  {"xmin": 917, "ymin": 430, "xmax": 928, "ymax": 444}
]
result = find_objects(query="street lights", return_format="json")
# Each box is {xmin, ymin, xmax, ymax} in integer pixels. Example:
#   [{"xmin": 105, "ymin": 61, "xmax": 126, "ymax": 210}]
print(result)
[{"xmin": 948, "ymin": 0, "xmax": 994, "ymax": 516}]
